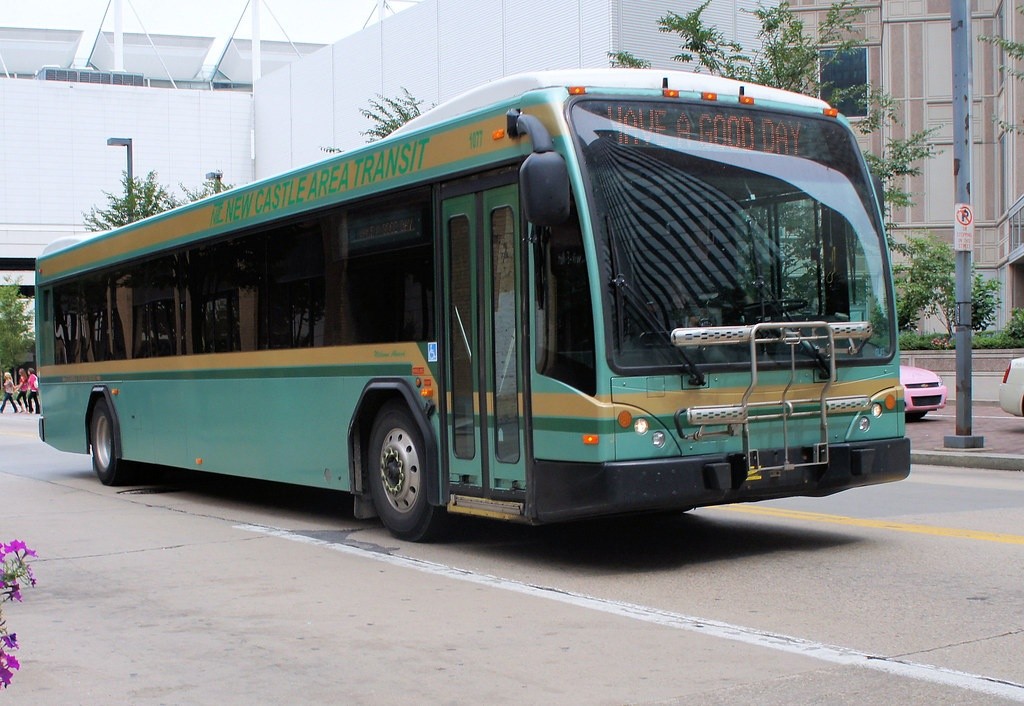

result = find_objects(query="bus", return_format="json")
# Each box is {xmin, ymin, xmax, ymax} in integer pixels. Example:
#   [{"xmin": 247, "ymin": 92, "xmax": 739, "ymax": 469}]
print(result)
[{"xmin": 28, "ymin": 62, "xmax": 915, "ymax": 550}]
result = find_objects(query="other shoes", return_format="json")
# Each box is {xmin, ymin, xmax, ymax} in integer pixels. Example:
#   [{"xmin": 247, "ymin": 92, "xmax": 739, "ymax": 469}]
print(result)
[
  {"xmin": 20, "ymin": 410, "xmax": 28, "ymax": 412},
  {"xmin": 29, "ymin": 410, "xmax": 40, "ymax": 414}
]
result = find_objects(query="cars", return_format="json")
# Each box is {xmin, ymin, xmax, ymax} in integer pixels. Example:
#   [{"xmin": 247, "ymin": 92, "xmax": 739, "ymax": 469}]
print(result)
[
  {"xmin": 897, "ymin": 362, "xmax": 949, "ymax": 423},
  {"xmin": 996, "ymin": 357, "xmax": 1024, "ymax": 422}
]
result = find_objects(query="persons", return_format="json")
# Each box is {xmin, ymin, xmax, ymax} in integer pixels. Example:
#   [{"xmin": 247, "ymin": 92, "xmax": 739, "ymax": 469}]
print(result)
[
  {"xmin": 25, "ymin": 368, "xmax": 42, "ymax": 413},
  {"xmin": 0, "ymin": 371, "xmax": 19, "ymax": 413},
  {"xmin": 647, "ymin": 232, "xmax": 723, "ymax": 332},
  {"xmin": 14, "ymin": 368, "xmax": 29, "ymax": 413}
]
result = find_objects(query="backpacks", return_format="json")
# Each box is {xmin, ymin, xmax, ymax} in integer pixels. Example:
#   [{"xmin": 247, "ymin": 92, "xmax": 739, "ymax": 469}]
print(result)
[{"xmin": 33, "ymin": 374, "xmax": 39, "ymax": 389}]
